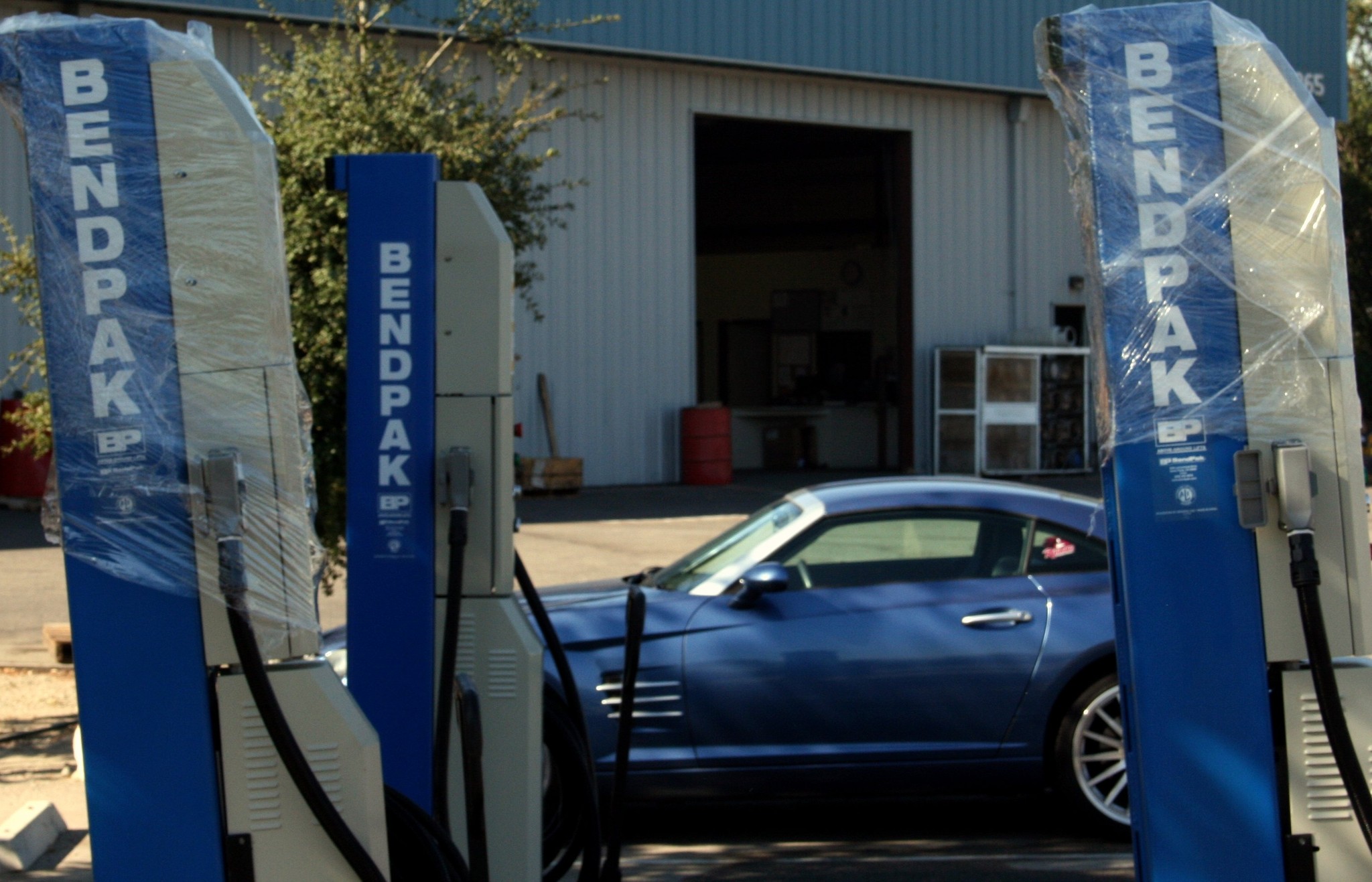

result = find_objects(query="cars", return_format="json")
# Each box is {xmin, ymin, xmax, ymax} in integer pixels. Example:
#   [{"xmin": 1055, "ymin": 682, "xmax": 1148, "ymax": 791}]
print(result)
[{"xmin": 317, "ymin": 475, "xmax": 1135, "ymax": 849}]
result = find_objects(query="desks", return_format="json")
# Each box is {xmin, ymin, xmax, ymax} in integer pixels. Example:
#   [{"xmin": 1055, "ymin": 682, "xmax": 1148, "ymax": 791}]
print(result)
[{"xmin": 729, "ymin": 402, "xmax": 878, "ymax": 472}]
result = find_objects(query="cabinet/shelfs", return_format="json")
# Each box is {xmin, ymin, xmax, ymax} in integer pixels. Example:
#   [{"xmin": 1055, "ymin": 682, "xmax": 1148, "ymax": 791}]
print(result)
[{"xmin": 929, "ymin": 344, "xmax": 1096, "ymax": 477}]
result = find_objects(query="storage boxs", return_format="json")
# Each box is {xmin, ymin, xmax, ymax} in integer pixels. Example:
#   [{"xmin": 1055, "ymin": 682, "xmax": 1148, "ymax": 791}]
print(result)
[{"xmin": 518, "ymin": 454, "xmax": 587, "ymax": 494}]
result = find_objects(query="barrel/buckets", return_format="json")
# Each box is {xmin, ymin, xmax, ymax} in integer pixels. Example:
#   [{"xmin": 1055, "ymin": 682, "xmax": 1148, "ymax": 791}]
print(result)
[{"xmin": 679, "ymin": 404, "xmax": 734, "ymax": 488}]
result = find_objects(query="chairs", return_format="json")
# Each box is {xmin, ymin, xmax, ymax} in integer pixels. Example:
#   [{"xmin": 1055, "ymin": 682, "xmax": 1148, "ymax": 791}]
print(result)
[{"xmin": 982, "ymin": 523, "xmax": 1023, "ymax": 573}]
